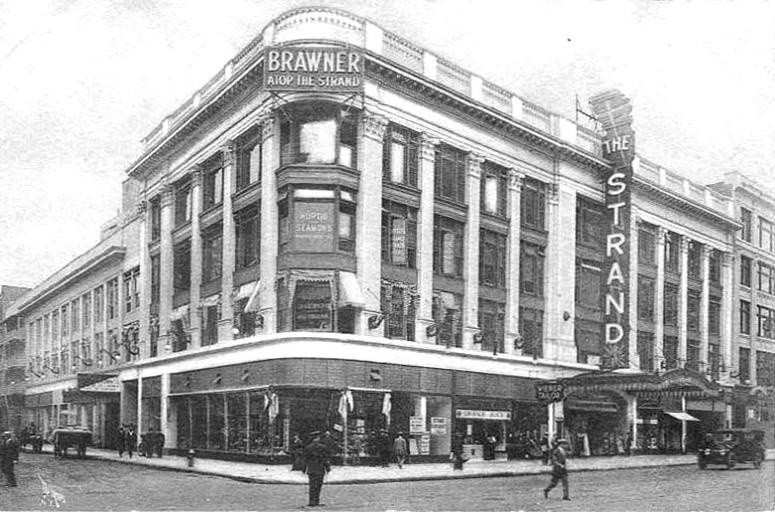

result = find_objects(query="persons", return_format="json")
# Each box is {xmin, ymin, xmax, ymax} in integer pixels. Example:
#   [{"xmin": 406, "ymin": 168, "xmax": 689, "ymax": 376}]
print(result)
[
  {"xmin": 381, "ymin": 428, "xmax": 393, "ymax": 467},
  {"xmin": 301, "ymin": 429, "xmax": 331, "ymax": 506},
  {"xmin": 395, "ymin": 429, "xmax": 408, "ymax": 468},
  {"xmin": 449, "ymin": 431, "xmax": 465, "ymax": 471},
  {"xmin": 323, "ymin": 431, "xmax": 334, "ymax": 474},
  {"xmin": 290, "ymin": 431, "xmax": 303, "ymax": 471},
  {"xmin": 154, "ymin": 428, "xmax": 165, "ymax": 459},
  {"xmin": 126, "ymin": 428, "xmax": 137, "ymax": 458},
  {"xmin": 143, "ymin": 426, "xmax": 154, "ymax": 457},
  {"xmin": 1, "ymin": 430, "xmax": 19, "ymax": 490},
  {"xmin": 543, "ymin": 438, "xmax": 572, "ymax": 502},
  {"xmin": 115, "ymin": 424, "xmax": 125, "ymax": 457}
]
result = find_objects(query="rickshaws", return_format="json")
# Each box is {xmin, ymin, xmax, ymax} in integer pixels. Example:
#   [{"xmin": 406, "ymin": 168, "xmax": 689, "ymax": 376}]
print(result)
[{"xmin": 50, "ymin": 426, "xmax": 92, "ymax": 459}]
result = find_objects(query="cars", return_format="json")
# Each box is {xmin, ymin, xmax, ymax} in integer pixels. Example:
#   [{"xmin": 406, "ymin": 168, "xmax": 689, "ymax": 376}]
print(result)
[{"xmin": 697, "ymin": 427, "xmax": 766, "ymax": 469}]
